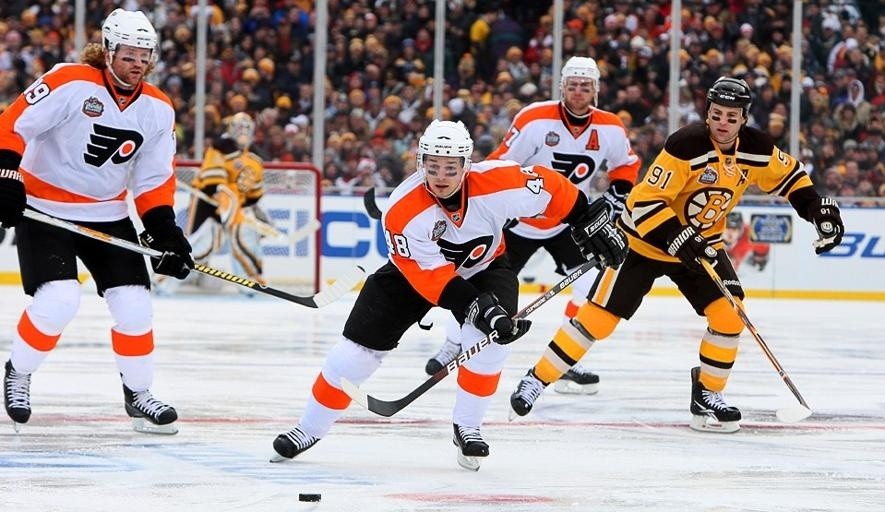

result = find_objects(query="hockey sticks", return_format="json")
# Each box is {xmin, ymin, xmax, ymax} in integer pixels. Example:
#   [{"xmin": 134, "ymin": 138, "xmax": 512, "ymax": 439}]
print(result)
[
  {"xmin": 700, "ymin": 257, "xmax": 813, "ymax": 423},
  {"xmin": 341, "ymin": 254, "xmax": 609, "ymax": 417},
  {"xmin": 176, "ymin": 179, "xmax": 320, "ymax": 245},
  {"xmin": 364, "ymin": 187, "xmax": 382, "ymax": 220},
  {"xmin": 23, "ymin": 207, "xmax": 365, "ymax": 308}
]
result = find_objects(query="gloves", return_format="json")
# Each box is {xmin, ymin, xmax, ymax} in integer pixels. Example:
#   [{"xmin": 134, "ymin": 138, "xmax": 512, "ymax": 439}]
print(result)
[
  {"xmin": 667, "ymin": 224, "xmax": 722, "ymax": 276},
  {"xmin": 601, "ymin": 180, "xmax": 634, "ymax": 223},
  {"xmin": 571, "ymin": 197, "xmax": 628, "ymax": 270},
  {"xmin": 0, "ymin": 165, "xmax": 26, "ymax": 228},
  {"xmin": 140, "ymin": 224, "xmax": 194, "ymax": 279},
  {"xmin": 807, "ymin": 197, "xmax": 844, "ymax": 254},
  {"xmin": 465, "ymin": 292, "xmax": 531, "ymax": 344}
]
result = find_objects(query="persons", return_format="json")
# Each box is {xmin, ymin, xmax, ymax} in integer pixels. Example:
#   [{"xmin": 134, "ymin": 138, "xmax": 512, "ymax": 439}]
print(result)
[
  {"xmin": 0, "ymin": 8, "xmax": 197, "ymax": 434},
  {"xmin": 424, "ymin": 55, "xmax": 644, "ymax": 397},
  {"xmin": 150, "ymin": 111, "xmax": 275, "ymax": 297},
  {"xmin": 270, "ymin": 119, "xmax": 633, "ymax": 471},
  {"xmin": 326, "ymin": 0, "xmax": 885, "ymax": 208},
  {"xmin": 1, "ymin": 1, "xmax": 121, "ymax": 110},
  {"xmin": 509, "ymin": 74, "xmax": 846, "ymax": 436},
  {"xmin": 122, "ymin": 0, "xmax": 314, "ymax": 164}
]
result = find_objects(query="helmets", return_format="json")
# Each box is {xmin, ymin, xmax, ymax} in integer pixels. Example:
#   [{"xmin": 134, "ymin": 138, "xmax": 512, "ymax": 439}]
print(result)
[
  {"xmin": 102, "ymin": 7, "xmax": 157, "ymax": 62},
  {"xmin": 560, "ymin": 55, "xmax": 600, "ymax": 92},
  {"xmin": 416, "ymin": 118, "xmax": 473, "ymax": 171},
  {"xmin": 705, "ymin": 76, "xmax": 752, "ymax": 116}
]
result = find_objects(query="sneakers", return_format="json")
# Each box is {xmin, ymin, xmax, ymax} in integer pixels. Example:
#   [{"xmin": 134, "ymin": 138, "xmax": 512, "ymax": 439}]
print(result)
[
  {"xmin": 454, "ymin": 423, "xmax": 489, "ymax": 456},
  {"xmin": 4, "ymin": 359, "xmax": 31, "ymax": 423},
  {"xmin": 273, "ymin": 424, "xmax": 320, "ymax": 458},
  {"xmin": 690, "ymin": 367, "xmax": 741, "ymax": 421},
  {"xmin": 559, "ymin": 363, "xmax": 599, "ymax": 385},
  {"xmin": 425, "ymin": 338, "xmax": 462, "ymax": 376},
  {"xmin": 123, "ymin": 383, "xmax": 177, "ymax": 425},
  {"xmin": 511, "ymin": 367, "xmax": 550, "ymax": 416}
]
